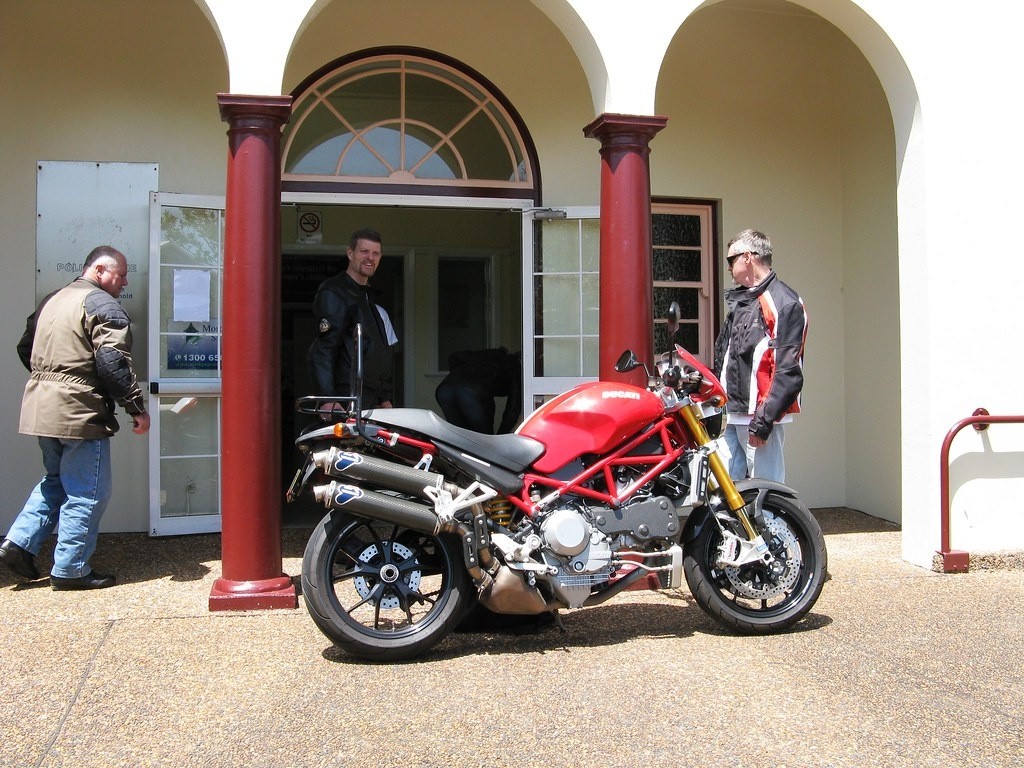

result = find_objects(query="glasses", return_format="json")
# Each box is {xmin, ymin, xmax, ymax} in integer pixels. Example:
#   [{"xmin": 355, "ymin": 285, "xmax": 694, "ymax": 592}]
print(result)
[{"xmin": 727, "ymin": 252, "xmax": 757, "ymax": 266}]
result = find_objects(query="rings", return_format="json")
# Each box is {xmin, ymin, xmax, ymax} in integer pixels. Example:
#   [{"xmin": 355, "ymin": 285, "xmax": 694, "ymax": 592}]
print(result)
[{"xmin": 323, "ymin": 417, "xmax": 327, "ymax": 420}]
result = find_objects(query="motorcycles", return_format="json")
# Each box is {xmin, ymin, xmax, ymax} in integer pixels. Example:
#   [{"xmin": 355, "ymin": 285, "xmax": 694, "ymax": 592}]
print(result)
[{"xmin": 286, "ymin": 301, "xmax": 827, "ymax": 663}]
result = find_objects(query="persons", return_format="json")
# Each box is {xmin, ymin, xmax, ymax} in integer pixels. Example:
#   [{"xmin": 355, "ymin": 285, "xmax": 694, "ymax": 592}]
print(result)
[
  {"xmin": 711, "ymin": 229, "xmax": 808, "ymax": 591},
  {"xmin": 0, "ymin": 244, "xmax": 149, "ymax": 591},
  {"xmin": 308, "ymin": 229, "xmax": 523, "ymax": 564}
]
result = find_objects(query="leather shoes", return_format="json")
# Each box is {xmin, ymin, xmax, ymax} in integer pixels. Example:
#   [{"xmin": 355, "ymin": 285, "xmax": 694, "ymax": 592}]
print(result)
[
  {"xmin": 51, "ymin": 570, "xmax": 116, "ymax": 591},
  {"xmin": 0, "ymin": 539, "xmax": 41, "ymax": 580}
]
